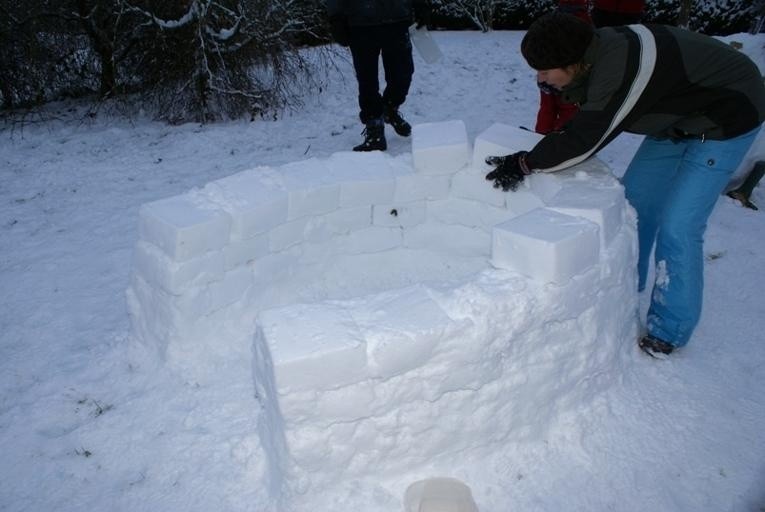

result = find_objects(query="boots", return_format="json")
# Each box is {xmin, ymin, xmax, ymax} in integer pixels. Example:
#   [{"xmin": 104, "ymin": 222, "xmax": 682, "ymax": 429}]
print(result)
[
  {"xmin": 382, "ymin": 97, "xmax": 412, "ymax": 136},
  {"xmin": 352, "ymin": 125, "xmax": 387, "ymax": 152}
]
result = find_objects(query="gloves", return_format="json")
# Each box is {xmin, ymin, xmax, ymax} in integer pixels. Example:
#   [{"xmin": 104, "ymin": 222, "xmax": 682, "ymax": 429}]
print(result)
[
  {"xmin": 326, "ymin": 11, "xmax": 354, "ymax": 47},
  {"xmin": 413, "ymin": 0, "xmax": 432, "ymax": 30},
  {"xmin": 484, "ymin": 151, "xmax": 531, "ymax": 193}
]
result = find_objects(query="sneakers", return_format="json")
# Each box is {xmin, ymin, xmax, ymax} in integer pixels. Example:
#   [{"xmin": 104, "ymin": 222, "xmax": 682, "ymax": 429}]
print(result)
[
  {"xmin": 726, "ymin": 160, "xmax": 764, "ymax": 202},
  {"xmin": 640, "ymin": 334, "xmax": 674, "ymax": 357}
]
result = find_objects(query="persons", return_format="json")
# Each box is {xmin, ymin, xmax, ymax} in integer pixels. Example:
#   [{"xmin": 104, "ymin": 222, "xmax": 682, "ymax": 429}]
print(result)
[{"xmin": 329, "ymin": 1, "xmax": 414, "ymax": 152}]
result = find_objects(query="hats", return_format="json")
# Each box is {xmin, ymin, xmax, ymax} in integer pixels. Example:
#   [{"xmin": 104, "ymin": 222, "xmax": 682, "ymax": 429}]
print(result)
[{"xmin": 520, "ymin": 12, "xmax": 591, "ymax": 72}]
